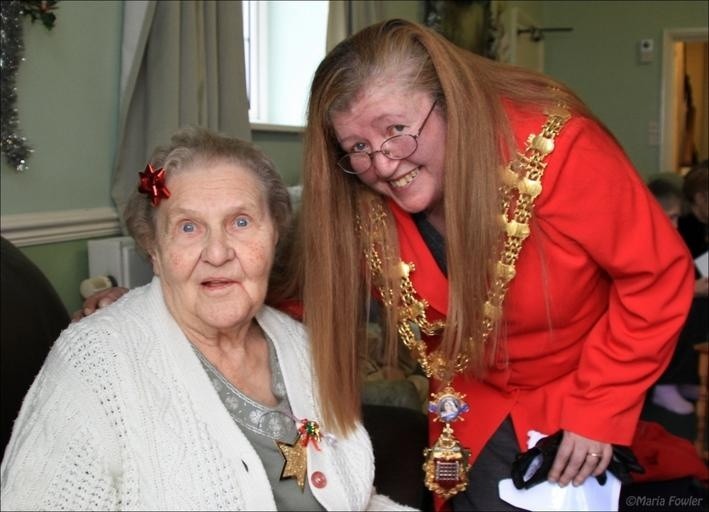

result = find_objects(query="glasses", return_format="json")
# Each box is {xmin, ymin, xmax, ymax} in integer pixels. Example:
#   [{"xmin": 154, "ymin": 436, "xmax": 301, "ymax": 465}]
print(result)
[{"xmin": 336, "ymin": 95, "xmax": 445, "ymax": 175}]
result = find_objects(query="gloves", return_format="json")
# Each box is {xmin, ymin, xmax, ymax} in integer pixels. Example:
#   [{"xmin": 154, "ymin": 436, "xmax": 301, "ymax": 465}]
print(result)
[{"xmin": 510, "ymin": 428, "xmax": 645, "ymax": 487}]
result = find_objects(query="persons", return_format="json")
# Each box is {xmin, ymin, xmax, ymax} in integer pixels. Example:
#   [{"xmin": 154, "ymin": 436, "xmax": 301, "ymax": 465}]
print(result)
[
  {"xmin": 0, "ymin": 123, "xmax": 422, "ymax": 512},
  {"xmin": 677, "ymin": 165, "xmax": 709, "ymax": 259},
  {"xmin": 67, "ymin": 16, "xmax": 696, "ymax": 512},
  {"xmin": 647, "ymin": 178, "xmax": 709, "ymax": 416}
]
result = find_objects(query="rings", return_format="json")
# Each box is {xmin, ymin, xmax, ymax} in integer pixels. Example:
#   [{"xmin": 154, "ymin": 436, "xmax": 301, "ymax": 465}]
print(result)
[{"xmin": 587, "ymin": 453, "xmax": 602, "ymax": 457}]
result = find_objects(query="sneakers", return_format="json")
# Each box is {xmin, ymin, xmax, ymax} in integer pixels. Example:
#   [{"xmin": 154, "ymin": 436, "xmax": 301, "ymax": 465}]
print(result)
[{"xmin": 653, "ymin": 384, "xmax": 694, "ymax": 415}]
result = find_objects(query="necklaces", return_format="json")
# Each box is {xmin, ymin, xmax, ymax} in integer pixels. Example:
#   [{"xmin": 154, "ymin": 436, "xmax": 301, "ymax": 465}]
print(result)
[{"xmin": 349, "ymin": 108, "xmax": 572, "ymax": 504}]
